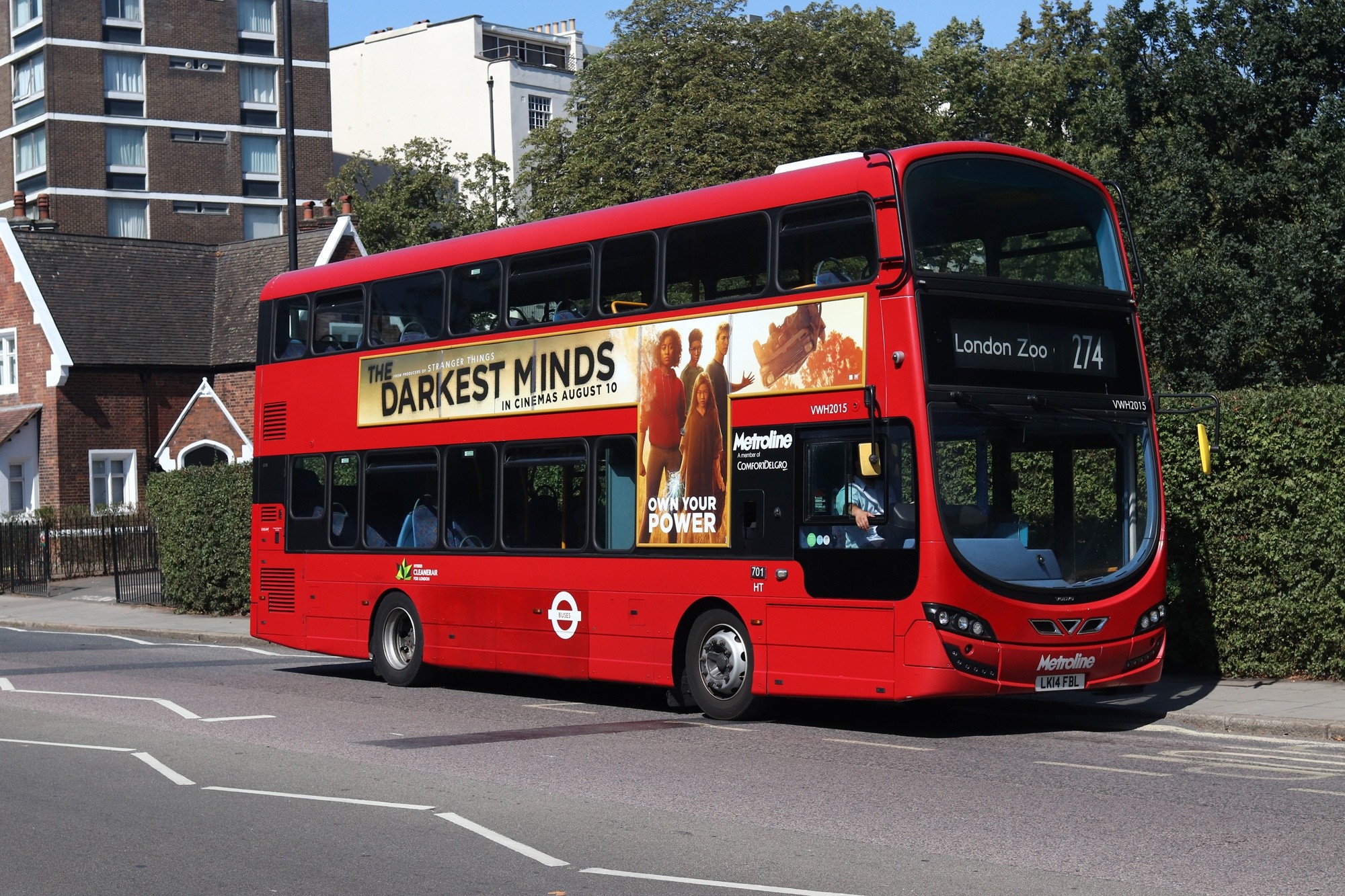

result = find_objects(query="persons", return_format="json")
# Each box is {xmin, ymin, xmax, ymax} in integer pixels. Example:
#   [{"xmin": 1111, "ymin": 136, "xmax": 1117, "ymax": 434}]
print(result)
[
  {"xmin": 835, "ymin": 448, "xmax": 897, "ymax": 532},
  {"xmin": 638, "ymin": 326, "xmax": 755, "ymax": 545}
]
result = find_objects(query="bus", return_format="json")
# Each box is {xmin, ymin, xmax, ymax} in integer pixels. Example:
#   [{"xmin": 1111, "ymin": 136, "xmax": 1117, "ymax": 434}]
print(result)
[{"xmin": 245, "ymin": 139, "xmax": 1222, "ymax": 723}]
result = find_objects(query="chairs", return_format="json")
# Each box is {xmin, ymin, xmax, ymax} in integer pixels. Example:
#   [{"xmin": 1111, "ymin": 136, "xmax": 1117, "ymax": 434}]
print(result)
[
  {"xmin": 860, "ymin": 262, "xmax": 878, "ymax": 280},
  {"xmin": 816, "ymin": 258, "xmax": 853, "ymax": 287},
  {"xmin": 286, "ymin": 485, "xmax": 592, "ymax": 551},
  {"xmin": 611, "ymin": 300, "xmax": 649, "ymax": 313},
  {"xmin": 275, "ymin": 298, "xmax": 586, "ymax": 361}
]
